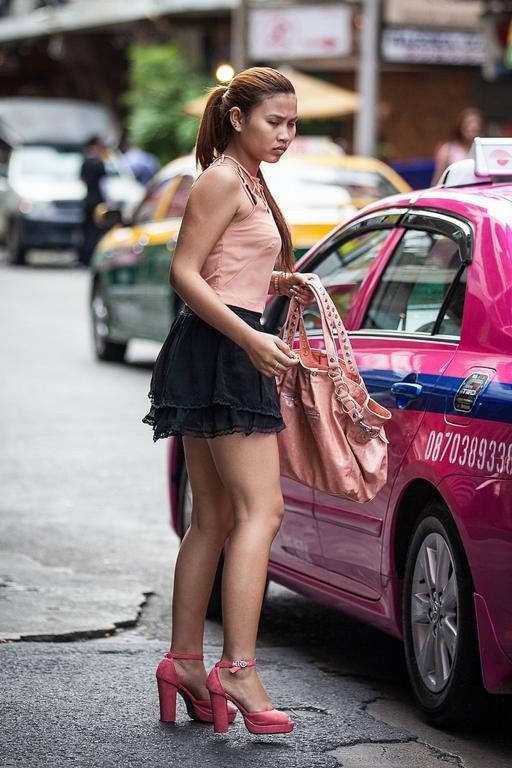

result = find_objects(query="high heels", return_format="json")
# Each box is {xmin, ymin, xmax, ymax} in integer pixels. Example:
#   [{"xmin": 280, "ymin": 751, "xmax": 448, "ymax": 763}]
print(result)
[
  {"xmin": 205, "ymin": 659, "xmax": 298, "ymax": 736},
  {"xmin": 156, "ymin": 652, "xmax": 241, "ymax": 727}
]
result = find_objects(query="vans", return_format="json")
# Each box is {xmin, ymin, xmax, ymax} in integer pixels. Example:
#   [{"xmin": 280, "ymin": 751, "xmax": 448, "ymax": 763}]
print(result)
[{"xmin": 0, "ymin": 92, "xmax": 147, "ymax": 262}]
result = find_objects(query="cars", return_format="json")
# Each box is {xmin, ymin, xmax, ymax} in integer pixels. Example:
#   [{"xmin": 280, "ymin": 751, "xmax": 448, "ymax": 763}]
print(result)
[
  {"xmin": 165, "ymin": 136, "xmax": 509, "ymax": 731},
  {"xmin": 87, "ymin": 137, "xmax": 417, "ymax": 364}
]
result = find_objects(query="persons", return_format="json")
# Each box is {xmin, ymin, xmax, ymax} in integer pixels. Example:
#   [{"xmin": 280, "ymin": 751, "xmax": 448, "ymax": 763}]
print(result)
[
  {"xmin": 145, "ymin": 67, "xmax": 316, "ymax": 733},
  {"xmin": 79, "ymin": 137, "xmax": 117, "ymax": 266},
  {"xmin": 429, "ymin": 104, "xmax": 484, "ymax": 188}
]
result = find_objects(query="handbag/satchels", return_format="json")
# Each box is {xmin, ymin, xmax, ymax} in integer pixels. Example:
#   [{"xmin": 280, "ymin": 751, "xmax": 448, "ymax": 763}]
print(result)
[{"xmin": 273, "ymin": 276, "xmax": 396, "ymax": 503}]
